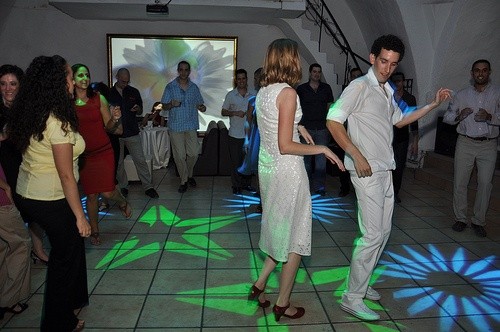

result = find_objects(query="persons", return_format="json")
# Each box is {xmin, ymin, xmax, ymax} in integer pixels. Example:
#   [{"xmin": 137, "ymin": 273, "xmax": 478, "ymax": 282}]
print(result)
[
  {"xmin": 443, "ymin": 59, "xmax": 500, "ymax": 237},
  {"xmin": 0, "ymin": 55, "xmax": 165, "ymax": 332},
  {"xmin": 221, "ymin": 35, "xmax": 453, "ymax": 321},
  {"xmin": 160, "ymin": 61, "xmax": 206, "ymax": 193}
]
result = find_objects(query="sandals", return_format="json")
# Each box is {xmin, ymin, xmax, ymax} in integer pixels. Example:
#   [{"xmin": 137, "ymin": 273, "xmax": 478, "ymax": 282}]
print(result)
[
  {"xmin": 89, "ymin": 229, "xmax": 101, "ymax": 246},
  {"xmin": 119, "ymin": 201, "xmax": 132, "ymax": 220}
]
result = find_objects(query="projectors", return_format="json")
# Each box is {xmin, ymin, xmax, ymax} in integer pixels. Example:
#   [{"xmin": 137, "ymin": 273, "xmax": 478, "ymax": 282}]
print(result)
[{"xmin": 146, "ymin": 4, "xmax": 170, "ymax": 16}]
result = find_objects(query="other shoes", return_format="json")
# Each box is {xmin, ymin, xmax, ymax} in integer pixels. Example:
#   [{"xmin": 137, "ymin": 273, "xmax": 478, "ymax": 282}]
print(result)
[
  {"xmin": 145, "ymin": 188, "xmax": 159, "ymax": 199},
  {"xmin": 394, "ymin": 194, "xmax": 401, "ymax": 203},
  {"xmin": 338, "ymin": 190, "xmax": 348, "ymax": 198},
  {"xmin": 243, "ymin": 184, "xmax": 252, "ymax": 190},
  {"xmin": 72, "ymin": 320, "xmax": 85, "ymax": 332},
  {"xmin": 316, "ymin": 190, "xmax": 327, "ymax": 194},
  {"xmin": 233, "ymin": 185, "xmax": 241, "ymax": 194},
  {"xmin": 452, "ymin": 221, "xmax": 466, "ymax": 232},
  {"xmin": 472, "ymin": 222, "xmax": 487, "ymax": 238},
  {"xmin": 179, "ymin": 183, "xmax": 187, "ymax": 194},
  {"xmin": 98, "ymin": 201, "xmax": 109, "ymax": 212},
  {"xmin": 120, "ymin": 187, "xmax": 128, "ymax": 197},
  {"xmin": 189, "ymin": 178, "xmax": 197, "ymax": 188}
]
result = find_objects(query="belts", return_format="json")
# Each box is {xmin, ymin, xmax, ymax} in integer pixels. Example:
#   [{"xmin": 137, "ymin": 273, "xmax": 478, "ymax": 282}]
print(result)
[{"xmin": 468, "ymin": 136, "xmax": 496, "ymax": 141}]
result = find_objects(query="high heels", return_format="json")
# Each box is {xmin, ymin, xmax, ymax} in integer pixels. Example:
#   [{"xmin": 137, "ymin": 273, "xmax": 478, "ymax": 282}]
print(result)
[
  {"xmin": 0, "ymin": 302, "xmax": 29, "ymax": 320},
  {"xmin": 273, "ymin": 302, "xmax": 305, "ymax": 321},
  {"xmin": 31, "ymin": 251, "xmax": 49, "ymax": 265},
  {"xmin": 249, "ymin": 285, "xmax": 270, "ymax": 308}
]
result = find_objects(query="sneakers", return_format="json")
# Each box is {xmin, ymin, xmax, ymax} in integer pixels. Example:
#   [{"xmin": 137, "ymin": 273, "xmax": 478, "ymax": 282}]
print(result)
[
  {"xmin": 364, "ymin": 286, "xmax": 381, "ymax": 300},
  {"xmin": 342, "ymin": 301, "xmax": 380, "ymax": 322}
]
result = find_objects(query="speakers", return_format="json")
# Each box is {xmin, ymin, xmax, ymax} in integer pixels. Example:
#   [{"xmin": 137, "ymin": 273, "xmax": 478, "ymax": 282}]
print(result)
[{"xmin": 434, "ymin": 117, "xmax": 458, "ymax": 156}]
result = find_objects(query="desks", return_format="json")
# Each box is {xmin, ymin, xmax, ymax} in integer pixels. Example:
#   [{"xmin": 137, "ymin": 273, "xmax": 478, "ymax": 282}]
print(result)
[{"xmin": 138, "ymin": 126, "xmax": 170, "ymax": 170}]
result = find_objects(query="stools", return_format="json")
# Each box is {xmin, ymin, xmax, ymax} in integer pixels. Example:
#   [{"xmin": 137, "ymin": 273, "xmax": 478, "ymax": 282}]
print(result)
[{"xmin": 124, "ymin": 155, "xmax": 154, "ymax": 183}]
